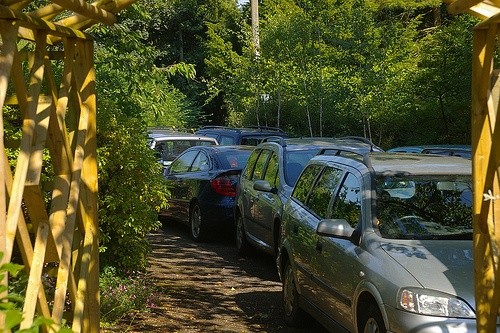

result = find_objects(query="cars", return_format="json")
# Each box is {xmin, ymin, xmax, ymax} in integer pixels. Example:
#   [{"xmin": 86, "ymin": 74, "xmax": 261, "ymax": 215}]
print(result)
[
  {"xmin": 386, "ymin": 146, "xmax": 472, "ymax": 160},
  {"xmin": 157, "ymin": 146, "xmax": 258, "ymax": 240}
]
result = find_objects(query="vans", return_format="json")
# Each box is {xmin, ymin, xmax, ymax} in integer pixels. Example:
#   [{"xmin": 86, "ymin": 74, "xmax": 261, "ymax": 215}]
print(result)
[{"xmin": 148, "ymin": 136, "xmax": 220, "ymax": 175}]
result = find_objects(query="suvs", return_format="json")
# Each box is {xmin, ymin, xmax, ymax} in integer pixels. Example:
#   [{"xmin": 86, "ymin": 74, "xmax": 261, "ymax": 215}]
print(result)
[
  {"xmin": 278, "ymin": 148, "xmax": 499, "ymax": 333},
  {"xmin": 193, "ymin": 125, "xmax": 288, "ymax": 146},
  {"xmin": 231, "ymin": 135, "xmax": 384, "ymax": 259}
]
